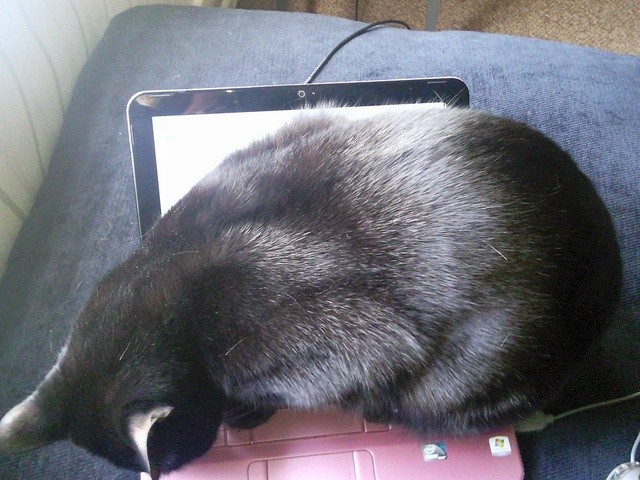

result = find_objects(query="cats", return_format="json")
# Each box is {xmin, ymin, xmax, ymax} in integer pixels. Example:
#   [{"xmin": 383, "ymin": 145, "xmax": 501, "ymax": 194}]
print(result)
[{"xmin": 0, "ymin": 90, "xmax": 623, "ymax": 480}]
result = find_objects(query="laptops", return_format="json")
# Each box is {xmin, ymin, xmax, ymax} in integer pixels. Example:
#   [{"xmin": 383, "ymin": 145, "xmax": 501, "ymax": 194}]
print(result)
[{"xmin": 125, "ymin": 77, "xmax": 526, "ymax": 479}]
